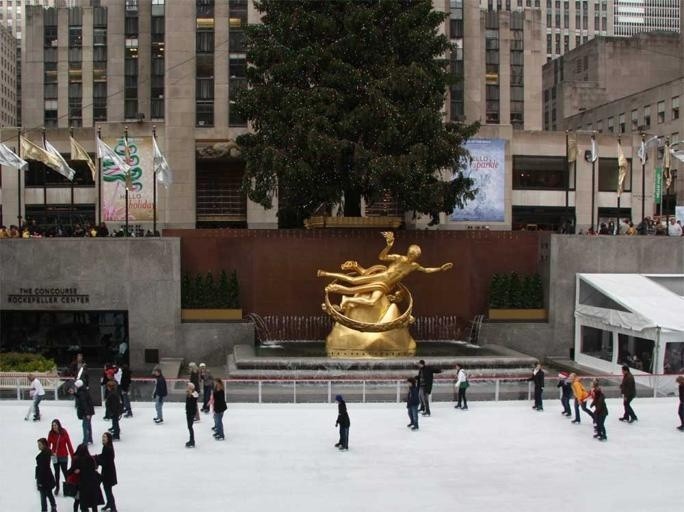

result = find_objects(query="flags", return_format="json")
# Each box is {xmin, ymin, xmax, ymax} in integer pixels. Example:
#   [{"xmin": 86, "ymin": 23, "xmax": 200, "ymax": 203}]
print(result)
[
  {"xmin": 569, "ymin": 133, "xmax": 681, "ymax": 197},
  {"xmin": 0, "ymin": 130, "xmax": 172, "ymax": 189}
]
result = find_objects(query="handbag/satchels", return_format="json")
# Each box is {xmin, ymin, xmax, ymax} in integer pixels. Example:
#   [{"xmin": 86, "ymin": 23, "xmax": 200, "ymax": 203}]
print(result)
[
  {"xmin": 51, "ymin": 453, "xmax": 57, "ymax": 463},
  {"xmin": 63, "ymin": 482, "xmax": 77, "ymax": 496}
]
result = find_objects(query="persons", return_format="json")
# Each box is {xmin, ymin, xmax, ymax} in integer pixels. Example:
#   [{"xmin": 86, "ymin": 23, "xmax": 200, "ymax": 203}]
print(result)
[
  {"xmin": 416, "ymin": 359, "xmax": 432, "ymax": 416},
  {"xmin": 150, "ymin": 366, "xmax": 167, "ymax": 423},
  {"xmin": 68, "ymin": 352, "xmax": 131, "ymax": 445},
  {"xmin": 184, "ymin": 361, "xmax": 226, "ymax": 446},
  {"xmin": 675, "ymin": 376, "xmax": 684, "ymax": 430},
  {"xmin": 530, "ymin": 361, "xmax": 608, "ymax": 440},
  {"xmin": 24, "ymin": 373, "xmax": 45, "ymax": 420},
  {"xmin": 315, "ymin": 232, "xmax": 457, "ymax": 310},
  {"xmin": 584, "ymin": 215, "xmax": 684, "ymax": 237},
  {"xmin": 616, "ymin": 367, "xmax": 638, "ymax": 424},
  {"xmin": 334, "ymin": 393, "xmax": 350, "ymax": 450},
  {"xmin": 406, "ymin": 378, "xmax": 418, "ymax": 431},
  {"xmin": 0, "ymin": 221, "xmax": 161, "ymax": 237},
  {"xmin": 33, "ymin": 419, "xmax": 122, "ymax": 512},
  {"xmin": 623, "ymin": 348, "xmax": 682, "ymax": 375},
  {"xmin": 454, "ymin": 365, "xmax": 468, "ymax": 411}
]
